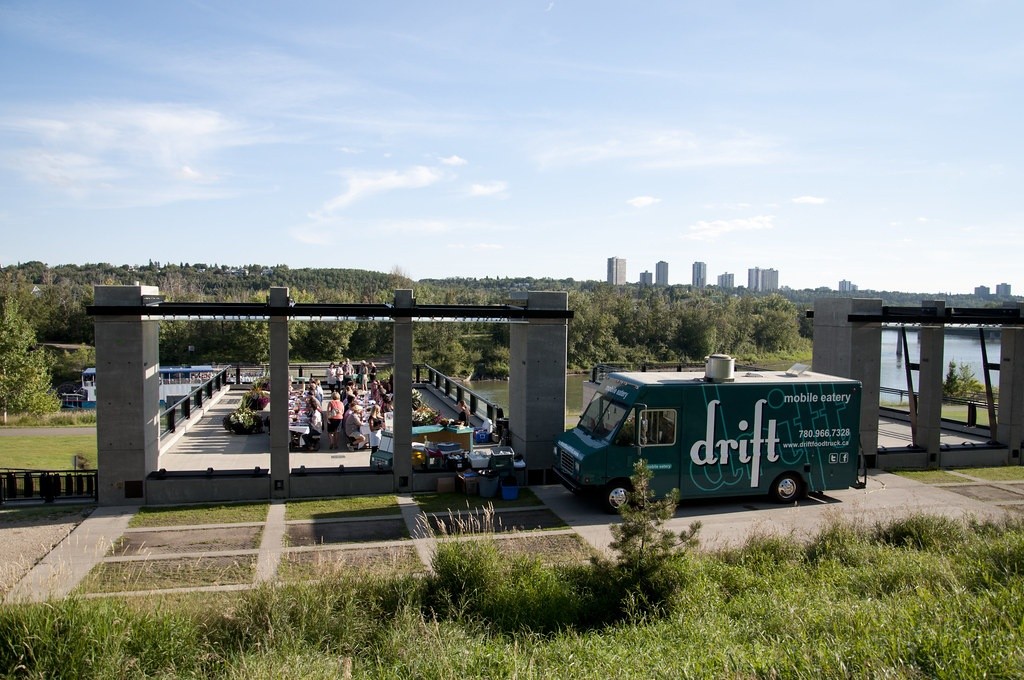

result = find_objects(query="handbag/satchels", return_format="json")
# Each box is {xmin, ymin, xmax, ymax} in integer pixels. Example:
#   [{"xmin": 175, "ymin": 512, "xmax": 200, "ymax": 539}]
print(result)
[
  {"xmin": 327, "ymin": 402, "xmax": 337, "ymax": 418},
  {"xmin": 356, "ymin": 374, "xmax": 362, "ymax": 384}
]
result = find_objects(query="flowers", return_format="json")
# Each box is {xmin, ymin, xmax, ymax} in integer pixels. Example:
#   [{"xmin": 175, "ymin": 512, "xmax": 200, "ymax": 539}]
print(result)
[
  {"xmin": 412, "ymin": 386, "xmax": 445, "ymax": 426},
  {"xmin": 223, "ymin": 376, "xmax": 271, "ymax": 436}
]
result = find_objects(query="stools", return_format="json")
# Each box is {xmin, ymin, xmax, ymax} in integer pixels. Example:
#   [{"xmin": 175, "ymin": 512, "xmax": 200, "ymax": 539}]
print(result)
[{"xmin": 310, "ymin": 435, "xmax": 322, "ymax": 451}]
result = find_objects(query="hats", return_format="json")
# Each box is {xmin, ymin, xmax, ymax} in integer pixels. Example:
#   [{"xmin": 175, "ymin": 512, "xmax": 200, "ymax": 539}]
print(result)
[
  {"xmin": 307, "ymin": 390, "xmax": 313, "ymax": 395},
  {"xmin": 359, "ymin": 360, "xmax": 366, "ymax": 367},
  {"xmin": 347, "ymin": 395, "xmax": 354, "ymax": 403}
]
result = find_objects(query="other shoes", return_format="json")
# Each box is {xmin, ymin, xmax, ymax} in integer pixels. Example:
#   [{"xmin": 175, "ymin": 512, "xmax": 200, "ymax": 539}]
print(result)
[
  {"xmin": 334, "ymin": 446, "xmax": 339, "ymax": 449},
  {"xmin": 306, "ymin": 448, "xmax": 315, "ymax": 452},
  {"xmin": 347, "ymin": 443, "xmax": 355, "ymax": 451},
  {"xmin": 329, "ymin": 443, "xmax": 333, "ymax": 450}
]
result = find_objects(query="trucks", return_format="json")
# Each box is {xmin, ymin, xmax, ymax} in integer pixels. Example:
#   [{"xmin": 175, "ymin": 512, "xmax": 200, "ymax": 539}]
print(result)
[
  {"xmin": 550, "ymin": 354, "xmax": 867, "ymax": 515},
  {"xmin": 370, "ymin": 425, "xmax": 473, "ymax": 471}
]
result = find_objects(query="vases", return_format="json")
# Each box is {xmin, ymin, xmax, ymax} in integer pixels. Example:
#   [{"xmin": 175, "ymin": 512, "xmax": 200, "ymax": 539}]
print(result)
[
  {"xmin": 235, "ymin": 426, "xmax": 254, "ymax": 435},
  {"xmin": 250, "ymin": 400, "xmax": 268, "ymax": 410},
  {"xmin": 260, "ymin": 383, "xmax": 271, "ymax": 391}
]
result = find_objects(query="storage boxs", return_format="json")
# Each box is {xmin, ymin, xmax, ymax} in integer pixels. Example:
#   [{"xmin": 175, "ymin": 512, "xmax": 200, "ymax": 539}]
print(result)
[
  {"xmin": 491, "ymin": 446, "xmax": 515, "ymax": 469},
  {"xmin": 477, "ymin": 432, "xmax": 489, "ymax": 443},
  {"xmin": 457, "ymin": 469, "xmax": 481, "ymax": 495},
  {"xmin": 466, "ymin": 452, "xmax": 490, "ymax": 469},
  {"xmin": 435, "ymin": 477, "xmax": 455, "ymax": 493}
]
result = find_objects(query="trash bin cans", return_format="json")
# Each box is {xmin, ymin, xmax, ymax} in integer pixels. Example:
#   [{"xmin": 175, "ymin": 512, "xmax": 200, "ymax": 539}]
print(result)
[{"xmin": 478, "ymin": 469, "xmax": 499, "ymax": 498}]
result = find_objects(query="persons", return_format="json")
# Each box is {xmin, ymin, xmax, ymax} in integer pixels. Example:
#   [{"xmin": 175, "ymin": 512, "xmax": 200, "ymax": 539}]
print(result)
[
  {"xmin": 453, "ymin": 401, "xmax": 470, "ymax": 420},
  {"xmin": 326, "ymin": 358, "xmax": 377, "ymax": 393},
  {"xmin": 341, "ymin": 381, "xmax": 393, "ymax": 451},
  {"xmin": 326, "ymin": 392, "xmax": 344, "ymax": 449},
  {"xmin": 192, "ymin": 372, "xmax": 263, "ymax": 383},
  {"xmin": 289, "ymin": 380, "xmax": 298, "ymax": 394},
  {"xmin": 300, "ymin": 378, "xmax": 323, "ymax": 452}
]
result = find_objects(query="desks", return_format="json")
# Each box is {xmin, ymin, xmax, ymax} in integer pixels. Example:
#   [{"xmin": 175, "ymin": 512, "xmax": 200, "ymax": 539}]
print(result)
[
  {"xmin": 290, "ymin": 385, "xmax": 311, "ymax": 450},
  {"xmin": 356, "ymin": 388, "xmax": 385, "ymax": 448}
]
